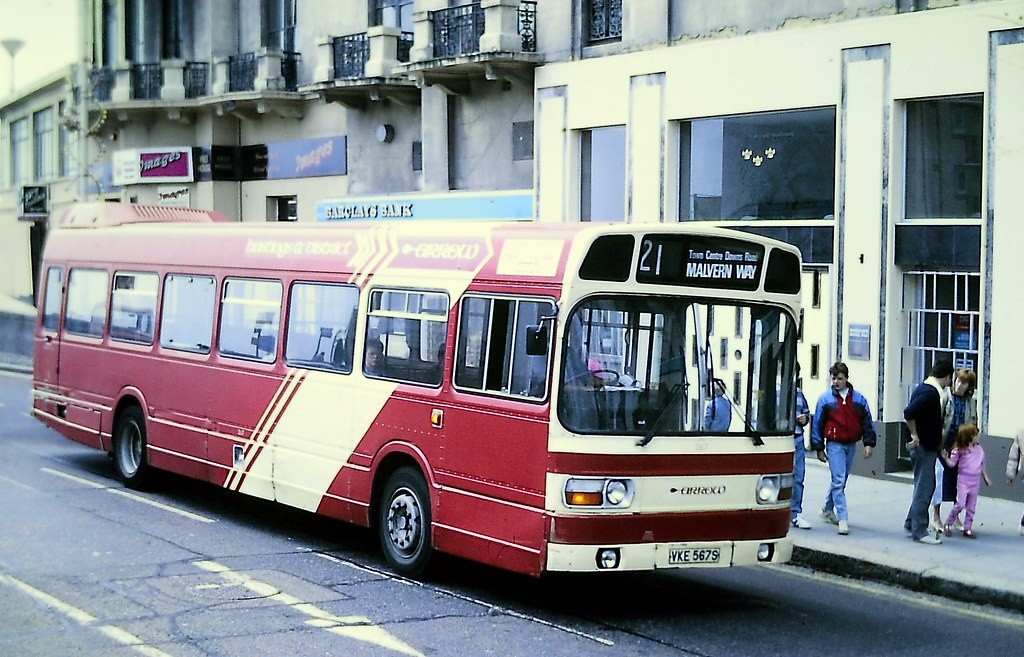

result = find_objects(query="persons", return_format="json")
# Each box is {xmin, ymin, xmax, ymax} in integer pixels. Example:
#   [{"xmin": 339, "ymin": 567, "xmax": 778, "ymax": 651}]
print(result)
[
  {"xmin": 931, "ymin": 369, "xmax": 979, "ymax": 533},
  {"xmin": 776, "ymin": 361, "xmax": 811, "ymax": 528},
  {"xmin": 701, "ymin": 378, "xmax": 731, "ymax": 431},
  {"xmin": 1005, "ymin": 427, "xmax": 1024, "ymax": 537},
  {"xmin": 810, "ymin": 362, "xmax": 877, "ymax": 535},
  {"xmin": 363, "ymin": 337, "xmax": 391, "ymax": 377},
  {"xmin": 426, "ymin": 342, "xmax": 446, "ymax": 385},
  {"xmin": 940, "ymin": 423, "xmax": 993, "ymax": 539},
  {"xmin": 903, "ymin": 359, "xmax": 955, "ymax": 543}
]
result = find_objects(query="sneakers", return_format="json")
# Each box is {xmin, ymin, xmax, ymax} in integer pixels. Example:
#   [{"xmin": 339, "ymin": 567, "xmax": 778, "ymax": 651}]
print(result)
[
  {"xmin": 921, "ymin": 535, "xmax": 942, "ymax": 545},
  {"xmin": 793, "ymin": 513, "xmax": 812, "ymax": 529},
  {"xmin": 819, "ymin": 509, "xmax": 838, "ymax": 524},
  {"xmin": 838, "ymin": 520, "xmax": 848, "ymax": 534}
]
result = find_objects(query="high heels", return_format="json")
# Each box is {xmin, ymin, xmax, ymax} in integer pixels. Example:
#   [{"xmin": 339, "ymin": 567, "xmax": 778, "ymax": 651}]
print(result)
[{"xmin": 932, "ymin": 520, "xmax": 964, "ymax": 531}]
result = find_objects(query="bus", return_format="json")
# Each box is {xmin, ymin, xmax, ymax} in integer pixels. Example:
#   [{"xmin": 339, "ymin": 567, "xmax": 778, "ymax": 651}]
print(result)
[{"xmin": 32, "ymin": 199, "xmax": 804, "ymax": 580}]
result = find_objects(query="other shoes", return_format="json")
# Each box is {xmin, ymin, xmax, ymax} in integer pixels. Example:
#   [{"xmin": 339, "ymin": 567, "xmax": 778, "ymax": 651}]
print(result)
[
  {"xmin": 945, "ymin": 524, "xmax": 952, "ymax": 537},
  {"xmin": 965, "ymin": 530, "xmax": 974, "ymax": 537}
]
